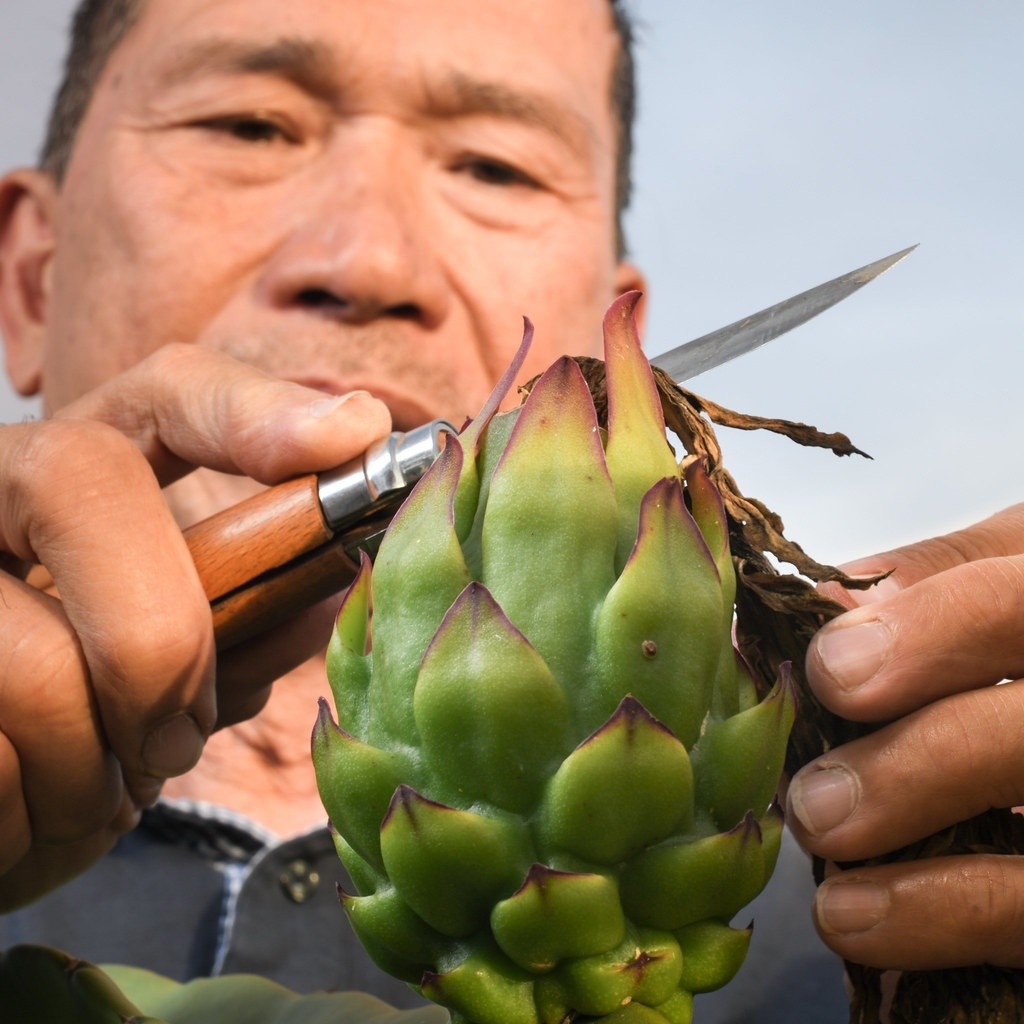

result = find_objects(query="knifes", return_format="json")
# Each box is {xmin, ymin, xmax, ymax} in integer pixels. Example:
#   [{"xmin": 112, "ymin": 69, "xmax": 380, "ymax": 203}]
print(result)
[{"xmin": 181, "ymin": 244, "xmax": 917, "ymax": 643}]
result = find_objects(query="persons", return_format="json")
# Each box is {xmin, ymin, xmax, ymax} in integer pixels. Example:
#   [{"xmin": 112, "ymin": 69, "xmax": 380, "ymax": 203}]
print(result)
[{"xmin": 0, "ymin": 0, "xmax": 1024, "ymax": 1024}]
